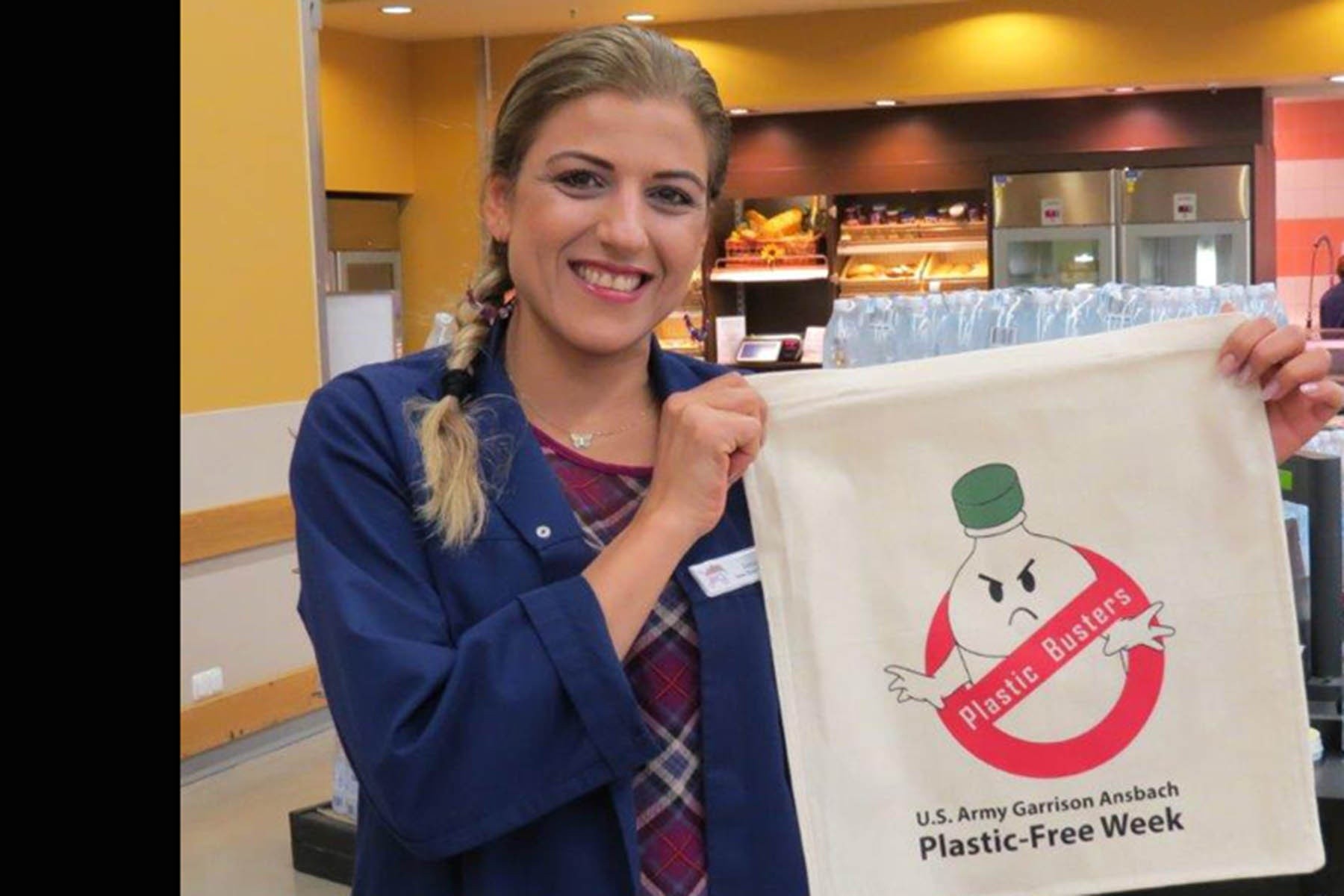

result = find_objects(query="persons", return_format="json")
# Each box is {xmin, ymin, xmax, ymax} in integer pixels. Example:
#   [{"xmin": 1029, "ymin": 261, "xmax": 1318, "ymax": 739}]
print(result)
[{"xmin": 288, "ymin": 26, "xmax": 1344, "ymax": 893}]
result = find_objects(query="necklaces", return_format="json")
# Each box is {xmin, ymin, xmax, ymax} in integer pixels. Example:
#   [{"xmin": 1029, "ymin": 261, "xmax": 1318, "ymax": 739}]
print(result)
[{"xmin": 506, "ymin": 369, "xmax": 657, "ymax": 450}]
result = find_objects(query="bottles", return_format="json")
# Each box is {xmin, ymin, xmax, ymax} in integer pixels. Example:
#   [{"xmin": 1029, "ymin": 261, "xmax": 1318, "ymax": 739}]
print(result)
[
  {"xmin": 843, "ymin": 200, "xmax": 980, "ymax": 225},
  {"xmin": 821, "ymin": 282, "xmax": 1290, "ymax": 370}
]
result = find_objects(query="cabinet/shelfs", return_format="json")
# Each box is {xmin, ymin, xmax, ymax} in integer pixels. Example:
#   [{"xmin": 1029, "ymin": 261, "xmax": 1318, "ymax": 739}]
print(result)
[{"xmin": 701, "ymin": 186, "xmax": 991, "ymax": 374}]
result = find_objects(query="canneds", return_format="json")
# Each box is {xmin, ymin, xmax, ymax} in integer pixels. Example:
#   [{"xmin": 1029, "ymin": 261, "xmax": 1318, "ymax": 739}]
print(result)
[{"xmin": 843, "ymin": 202, "xmax": 985, "ymax": 226}]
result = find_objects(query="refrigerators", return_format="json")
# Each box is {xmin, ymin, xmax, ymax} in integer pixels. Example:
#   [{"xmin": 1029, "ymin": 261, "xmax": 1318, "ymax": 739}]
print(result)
[{"xmin": 981, "ymin": 143, "xmax": 1253, "ymax": 286}]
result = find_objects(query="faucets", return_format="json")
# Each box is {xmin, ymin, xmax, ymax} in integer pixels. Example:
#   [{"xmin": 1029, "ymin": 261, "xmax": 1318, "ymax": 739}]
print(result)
[{"xmin": 1306, "ymin": 234, "xmax": 1334, "ymax": 331}]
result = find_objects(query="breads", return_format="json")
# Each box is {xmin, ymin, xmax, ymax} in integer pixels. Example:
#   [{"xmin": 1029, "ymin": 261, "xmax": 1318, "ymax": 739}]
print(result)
[
  {"xmin": 846, "ymin": 251, "xmax": 987, "ymax": 279},
  {"xmin": 724, "ymin": 207, "xmax": 804, "ymax": 239}
]
